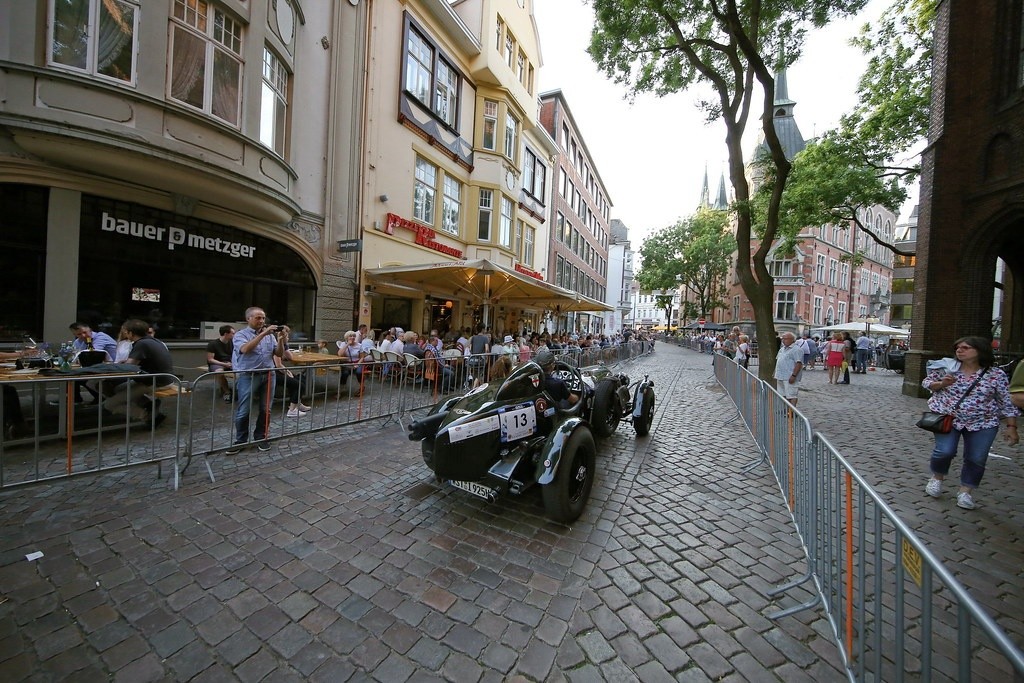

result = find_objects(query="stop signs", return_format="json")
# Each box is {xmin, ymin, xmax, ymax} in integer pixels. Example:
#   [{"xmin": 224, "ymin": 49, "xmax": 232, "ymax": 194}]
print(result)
[{"xmin": 699, "ymin": 317, "xmax": 706, "ymax": 324}]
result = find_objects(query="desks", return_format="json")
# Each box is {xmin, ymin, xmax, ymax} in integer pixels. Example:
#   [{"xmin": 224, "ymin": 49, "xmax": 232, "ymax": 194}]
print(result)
[
  {"xmin": 0, "ymin": 355, "xmax": 146, "ymax": 447},
  {"xmin": 275, "ymin": 351, "xmax": 349, "ymax": 403}
]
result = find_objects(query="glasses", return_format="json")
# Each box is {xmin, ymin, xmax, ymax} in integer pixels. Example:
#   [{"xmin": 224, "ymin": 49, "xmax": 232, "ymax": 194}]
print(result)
[
  {"xmin": 955, "ymin": 345, "xmax": 975, "ymax": 352},
  {"xmin": 781, "ymin": 337, "xmax": 793, "ymax": 340}
]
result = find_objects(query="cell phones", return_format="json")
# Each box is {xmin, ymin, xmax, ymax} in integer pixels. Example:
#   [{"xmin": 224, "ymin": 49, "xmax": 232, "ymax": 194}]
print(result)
[{"xmin": 273, "ymin": 326, "xmax": 284, "ymax": 333}]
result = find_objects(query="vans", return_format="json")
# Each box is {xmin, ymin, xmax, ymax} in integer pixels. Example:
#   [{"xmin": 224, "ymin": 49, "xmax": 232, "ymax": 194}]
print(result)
[{"xmin": 991, "ymin": 320, "xmax": 1003, "ymax": 355}]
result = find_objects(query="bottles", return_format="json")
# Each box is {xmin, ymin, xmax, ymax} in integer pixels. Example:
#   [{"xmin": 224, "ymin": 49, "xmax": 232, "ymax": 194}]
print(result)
[
  {"xmin": 59, "ymin": 340, "xmax": 73, "ymax": 373},
  {"xmin": 85, "ymin": 337, "xmax": 93, "ymax": 351}
]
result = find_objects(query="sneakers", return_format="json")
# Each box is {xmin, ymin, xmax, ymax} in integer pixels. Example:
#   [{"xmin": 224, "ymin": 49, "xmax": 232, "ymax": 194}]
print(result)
[
  {"xmin": 299, "ymin": 404, "xmax": 312, "ymax": 411},
  {"xmin": 926, "ymin": 475, "xmax": 942, "ymax": 497},
  {"xmin": 287, "ymin": 408, "xmax": 307, "ymax": 417},
  {"xmin": 957, "ymin": 491, "xmax": 975, "ymax": 509}
]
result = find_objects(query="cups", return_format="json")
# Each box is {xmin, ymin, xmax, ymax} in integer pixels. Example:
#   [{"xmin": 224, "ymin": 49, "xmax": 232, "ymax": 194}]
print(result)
[
  {"xmin": 298, "ymin": 344, "xmax": 303, "ymax": 352},
  {"xmin": 307, "ymin": 346, "xmax": 311, "ymax": 353},
  {"xmin": 15, "ymin": 343, "xmax": 25, "ymax": 359}
]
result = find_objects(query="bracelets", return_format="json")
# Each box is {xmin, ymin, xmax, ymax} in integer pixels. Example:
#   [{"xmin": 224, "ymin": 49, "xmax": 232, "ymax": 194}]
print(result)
[{"xmin": 1007, "ymin": 424, "xmax": 1018, "ymax": 429}]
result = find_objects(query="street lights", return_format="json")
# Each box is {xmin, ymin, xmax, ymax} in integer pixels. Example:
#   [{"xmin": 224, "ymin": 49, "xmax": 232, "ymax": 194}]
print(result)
[
  {"xmin": 804, "ymin": 320, "xmax": 812, "ymax": 338},
  {"xmin": 857, "ymin": 313, "xmax": 880, "ymax": 337},
  {"xmin": 901, "ymin": 321, "xmax": 911, "ymax": 346}
]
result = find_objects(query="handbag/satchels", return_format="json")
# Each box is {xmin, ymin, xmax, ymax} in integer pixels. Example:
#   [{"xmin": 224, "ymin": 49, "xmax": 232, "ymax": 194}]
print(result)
[{"xmin": 916, "ymin": 411, "xmax": 953, "ymax": 434}]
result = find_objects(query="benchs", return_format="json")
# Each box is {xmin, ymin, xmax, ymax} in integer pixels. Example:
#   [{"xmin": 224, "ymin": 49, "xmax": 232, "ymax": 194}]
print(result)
[
  {"xmin": 330, "ymin": 366, "xmax": 372, "ymax": 375},
  {"xmin": 198, "ymin": 366, "xmax": 240, "ymax": 379},
  {"xmin": 147, "ymin": 385, "xmax": 190, "ymax": 398}
]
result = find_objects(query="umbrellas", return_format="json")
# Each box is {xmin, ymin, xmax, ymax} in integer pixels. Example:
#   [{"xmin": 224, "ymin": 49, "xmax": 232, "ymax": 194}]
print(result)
[
  {"xmin": 809, "ymin": 319, "xmax": 911, "ymax": 337},
  {"xmin": 677, "ymin": 320, "xmax": 730, "ymax": 334},
  {"xmin": 366, "ymin": 259, "xmax": 615, "ymax": 332}
]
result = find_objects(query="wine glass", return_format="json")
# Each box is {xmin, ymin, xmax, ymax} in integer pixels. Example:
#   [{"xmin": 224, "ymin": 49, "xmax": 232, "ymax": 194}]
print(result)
[{"xmin": 40, "ymin": 348, "xmax": 53, "ymax": 368}]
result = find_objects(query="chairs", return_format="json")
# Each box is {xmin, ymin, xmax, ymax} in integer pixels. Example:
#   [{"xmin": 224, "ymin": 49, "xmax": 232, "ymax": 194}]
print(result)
[{"xmin": 336, "ymin": 340, "xmax": 464, "ymax": 395}]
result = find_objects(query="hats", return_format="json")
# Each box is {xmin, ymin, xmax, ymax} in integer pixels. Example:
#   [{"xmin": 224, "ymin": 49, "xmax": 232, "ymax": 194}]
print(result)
[
  {"xmin": 504, "ymin": 335, "xmax": 514, "ymax": 344},
  {"xmin": 537, "ymin": 351, "xmax": 555, "ymax": 367}
]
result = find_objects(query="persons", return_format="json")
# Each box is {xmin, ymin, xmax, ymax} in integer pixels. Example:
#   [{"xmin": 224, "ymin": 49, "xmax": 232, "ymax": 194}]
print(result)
[
  {"xmin": 655, "ymin": 325, "xmax": 750, "ymax": 369},
  {"xmin": 102, "ymin": 319, "xmax": 175, "ymax": 430},
  {"xmin": 920, "ymin": 337, "xmax": 1024, "ymax": 510},
  {"xmin": 317, "ymin": 339, "xmax": 330, "ymax": 354},
  {"xmin": 270, "ymin": 322, "xmax": 311, "ymax": 416},
  {"xmin": 358, "ymin": 322, "xmax": 656, "ymax": 397},
  {"xmin": 851, "ymin": 332, "xmax": 868, "ymax": 374},
  {"xmin": 796, "ymin": 335, "xmax": 888, "ymax": 367},
  {"xmin": 355, "ymin": 324, "xmax": 367, "ymax": 343},
  {"xmin": 774, "ymin": 331, "xmax": 782, "ymax": 351},
  {"xmin": 536, "ymin": 350, "xmax": 579, "ymax": 403},
  {"xmin": 335, "ymin": 330, "xmax": 365, "ymax": 399},
  {"xmin": 49, "ymin": 322, "xmax": 116, "ymax": 405},
  {"xmin": 825, "ymin": 331, "xmax": 857, "ymax": 385},
  {"xmin": 0, "ymin": 348, "xmax": 39, "ymax": 437},
  {"xmin": 223, "ymin": 307, "xmax": 289, "ymax": 455},
  {"xmin": 771, "ymin": 333, "xmax": 803, "ymax": 418},
  {"xmin": 205, "ymin": 325, "xmax": 237, "ymax": 404}
]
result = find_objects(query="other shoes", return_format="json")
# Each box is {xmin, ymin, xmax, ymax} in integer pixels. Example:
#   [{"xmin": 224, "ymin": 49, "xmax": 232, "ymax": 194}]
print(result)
[
  {"xmin": 355, "ymin": 386, "xmax": 365, "ymax": 396},
  {"xmin": 777, "ymin": 409, "xmax": 788, "ymax": 414},
  {"xmin": 88, "ymin": 396, "xmax": 108, "ymax": 406},
  {"xmin": 855, "ymin": 371, "xmax": 860, "ymax": 374},
  {"xmin": 226, "ymin": 441, "xmax": 244, "ymax": 454},
  {"xmin": 784, "ymin": 412, "xmax": 797, "ymax": 418},
  {"xmin": 49, "ymin": 394, "xmax": 84, "ymax": 405},
  {"xmin": 139, "ymin": 414, "xmax": 166, "ymax": 431},
  {"xmin": 840, "ymin": 380, "xmax": 849, "ymax": 384},
  {"xmin": 255, "ymin": 439, "xmax": 271, "ymax": 451},
  {"xmin": 861, "ymin": 371, "xmax": 867, "ymax": 374},
  {"xmin": 224, "ymin": 388, "xmax": 233, "ymax": 404},
  {"xmin": 439, "ymin": 386, "xmax": 454, "ymax": 395},
  {"xmin": 828, "ymin": 381, "xmax": 832, "ymax": 384},
  {"xmin": 833, "ymin": 381, "xmax": 837, "ymax": 385}
]
